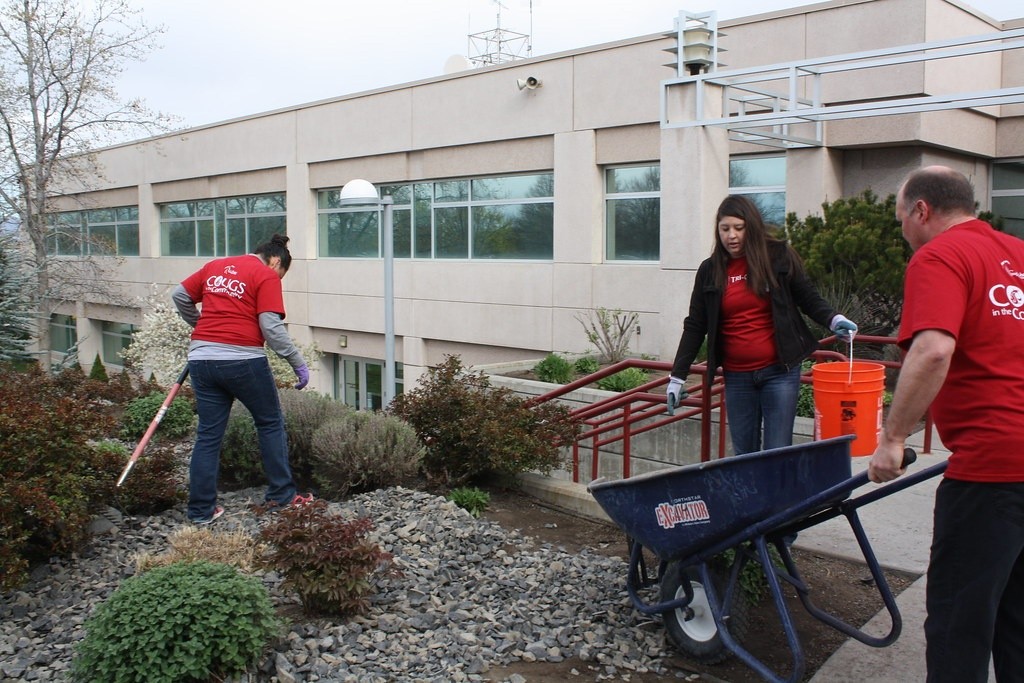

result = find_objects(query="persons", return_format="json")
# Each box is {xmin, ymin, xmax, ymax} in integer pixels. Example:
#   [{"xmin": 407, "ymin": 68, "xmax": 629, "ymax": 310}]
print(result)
[
  {"xmin": 171, "ymin": 234, "xmax": 315, "ymax": 525},
  {"xmin": 664, "ymin": 194, "xmax": 858, "ymax": 549},
  {"xmin": 864, "ymin": 164, "xmax": 1023, "ymax": 683}
]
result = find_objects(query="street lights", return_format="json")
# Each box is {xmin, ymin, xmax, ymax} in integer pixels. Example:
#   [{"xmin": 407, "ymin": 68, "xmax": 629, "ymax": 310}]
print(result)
[{"xmin": 334, "ymin": 178, "xmax": 398, "ymax": 413}]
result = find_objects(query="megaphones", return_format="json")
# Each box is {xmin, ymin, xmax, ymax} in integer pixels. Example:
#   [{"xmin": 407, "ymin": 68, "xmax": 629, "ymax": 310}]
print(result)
[
  {"xmin": 526, "ymin": 77, "xmax": 539, "ymax": 89},
  {"xmin": 517, "ymin": 79, "xmax": 527, "ymax": 90}
]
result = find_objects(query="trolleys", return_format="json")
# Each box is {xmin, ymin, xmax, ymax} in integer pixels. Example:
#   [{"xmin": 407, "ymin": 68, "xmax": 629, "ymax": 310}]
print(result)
[{"xmin": 587, "ymin": 432, "xmax": 950, "ymax": 683}]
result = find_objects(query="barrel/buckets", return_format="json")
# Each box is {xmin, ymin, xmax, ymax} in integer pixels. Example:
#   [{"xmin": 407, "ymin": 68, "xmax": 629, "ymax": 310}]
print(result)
[{"xmin": 812, "ymin": 362, "xmax": 887, "ymax": 457}]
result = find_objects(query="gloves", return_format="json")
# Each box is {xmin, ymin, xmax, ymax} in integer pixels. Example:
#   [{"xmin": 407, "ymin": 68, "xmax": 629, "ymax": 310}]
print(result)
[
  {"xmin": 294, "ymin": 363, "xmax": 310, "ymax": 390},
  {"xmin": 830, "ymin": 314, "xmax": 858, "ymax": 343},
  {"xmin": 666, "ymin": 377, "xmax": 688, "ymax": 416}
]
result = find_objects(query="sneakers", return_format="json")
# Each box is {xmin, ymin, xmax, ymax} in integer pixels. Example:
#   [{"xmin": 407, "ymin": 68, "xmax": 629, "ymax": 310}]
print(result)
[
  {"xmin": 286, "ymin": 492, "xmax": 315, "ymax": 507},
  {"xmin": 191, "ymin": 504, "xmax": 224, "ymax": 525}
]
